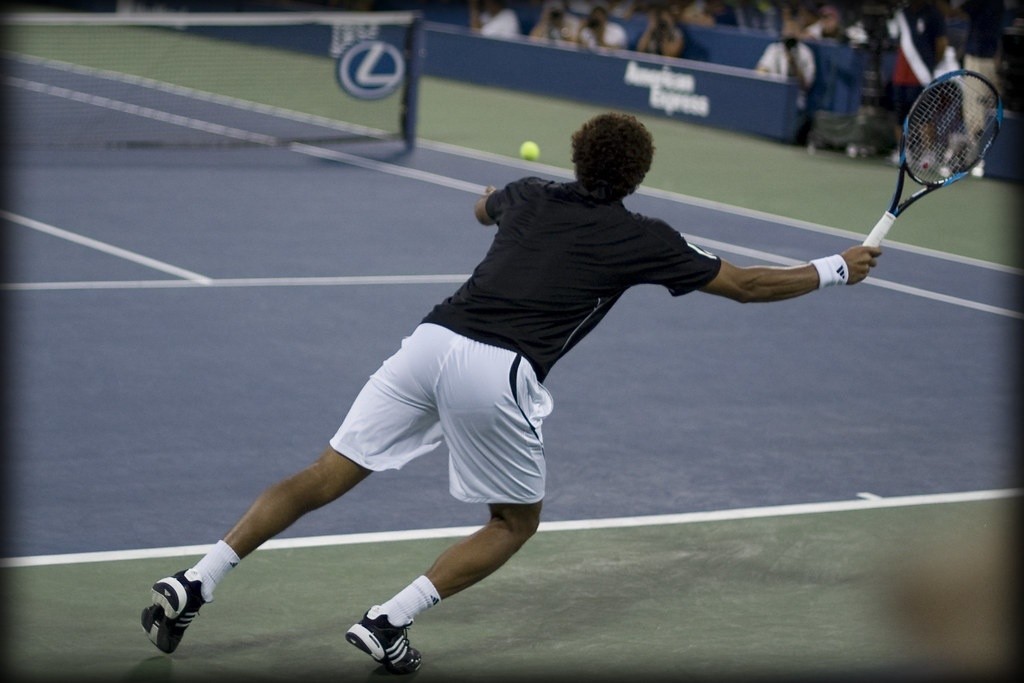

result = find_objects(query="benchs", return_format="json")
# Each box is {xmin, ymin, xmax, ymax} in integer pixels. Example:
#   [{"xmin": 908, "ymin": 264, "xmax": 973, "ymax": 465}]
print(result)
[{"xmin": 421, "ymin": 0, "xmax": 898, "ymax": 123}]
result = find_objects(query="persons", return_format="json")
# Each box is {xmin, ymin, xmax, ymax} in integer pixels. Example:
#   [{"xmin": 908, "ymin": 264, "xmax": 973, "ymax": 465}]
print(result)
[
  {"xmin": 142, "ymin": 114, "xmax": 883, "ymax": 676},
  {"xmin": 465, "ymin": 0, "xmax": 1024, "ymax": 178}
]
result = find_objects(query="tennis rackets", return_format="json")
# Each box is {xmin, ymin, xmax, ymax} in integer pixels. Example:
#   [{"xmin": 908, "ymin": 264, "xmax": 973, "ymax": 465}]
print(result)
[{"xmin": 862, "ymin": 69, "xmax": 1002, "ymax": 247}]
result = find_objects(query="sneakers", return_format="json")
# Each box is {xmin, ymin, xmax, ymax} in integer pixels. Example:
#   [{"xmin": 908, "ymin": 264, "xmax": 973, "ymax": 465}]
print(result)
[
  {"xmin": 140, "ymin": 567, "xmax": 214, "ymax": 654},
  {"xmin": 344, "ymin": 605, "xmax": 423, "ymax": 675}
]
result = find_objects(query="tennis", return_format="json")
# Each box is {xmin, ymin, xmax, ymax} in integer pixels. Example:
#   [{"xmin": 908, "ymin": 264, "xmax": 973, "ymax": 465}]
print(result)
[{"xmin": 518, "ymin": 140, "xmax": 540, "ymax": 161}]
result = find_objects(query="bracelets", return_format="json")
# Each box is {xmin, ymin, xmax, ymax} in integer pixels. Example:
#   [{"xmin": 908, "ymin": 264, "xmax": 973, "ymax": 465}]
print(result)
[{"xmin": 810, "ymin": 254, "xmax": 848, "ymax": 291}]
